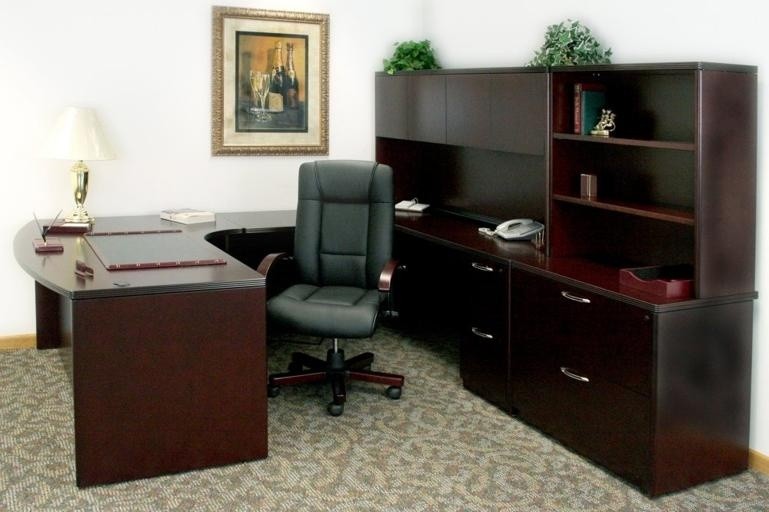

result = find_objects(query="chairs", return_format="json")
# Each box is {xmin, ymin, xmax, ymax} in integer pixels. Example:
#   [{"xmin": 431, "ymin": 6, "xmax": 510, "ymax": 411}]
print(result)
[{"xmin": 256, "ymin": 159, "xmax": 407, "ymax": 416}]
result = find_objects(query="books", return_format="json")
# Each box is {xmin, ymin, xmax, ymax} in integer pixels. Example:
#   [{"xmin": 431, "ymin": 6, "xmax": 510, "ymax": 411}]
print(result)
[{"xmin": 160, "ymin": 208, "xmax": 216, "ymax": 225}]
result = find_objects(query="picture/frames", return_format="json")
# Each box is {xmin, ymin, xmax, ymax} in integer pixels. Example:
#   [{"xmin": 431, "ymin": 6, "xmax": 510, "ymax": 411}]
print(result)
[{"xmin": 210, "ymin": 5, "xmax": 331, "ymax": 157}]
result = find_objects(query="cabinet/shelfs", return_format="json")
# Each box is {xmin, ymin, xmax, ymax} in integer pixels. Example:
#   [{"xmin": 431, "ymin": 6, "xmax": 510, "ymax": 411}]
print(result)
[
  {"xmin": 445, "ymin": 67, "xmax": 548, "ymax": 153},
  {"xmin": 509, "ymin": 261, "xmax": 752, "ymax": 498},
  {"xmin": 548, "ymin": 63, "xmax": 758, "ymax": 299},
  {"xmin": 460, "ymin": 253, "xmax": 510, "ymax": 418},
  {"xmin": 377, "ymin": 70, "xmax": 445, "ymax": 145}
]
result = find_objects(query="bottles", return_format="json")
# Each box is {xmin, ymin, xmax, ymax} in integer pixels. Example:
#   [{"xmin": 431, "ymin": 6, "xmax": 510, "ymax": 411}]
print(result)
[
  {"xmin": 285, "ymin": 42, "xmax": 298, "ymax": 109},
  {"xmin": 270, "ymin": 42, "xmax": 285, "ymax": 115}
]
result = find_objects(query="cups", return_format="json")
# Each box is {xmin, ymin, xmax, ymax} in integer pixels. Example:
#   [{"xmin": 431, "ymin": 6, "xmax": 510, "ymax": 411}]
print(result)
[{"xmin": 579, "ymin": 173, "xmax": 600, "ymax": 200}]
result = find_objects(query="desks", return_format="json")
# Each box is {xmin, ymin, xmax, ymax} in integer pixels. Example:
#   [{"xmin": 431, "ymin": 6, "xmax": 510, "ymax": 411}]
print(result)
[{"xmin": 11, "ymin": 200, "xmax": 296, "ymax": 490}]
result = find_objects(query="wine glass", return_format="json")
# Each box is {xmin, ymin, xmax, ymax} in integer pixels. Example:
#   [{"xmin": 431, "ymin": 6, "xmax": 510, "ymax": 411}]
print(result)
[
  {"xmin": 255, "ymin": 74, "xmax": 270, "ymax": 121},
  {"xmin": 249, "ymin": 70, "xmax": 263, "ymax": 114}
]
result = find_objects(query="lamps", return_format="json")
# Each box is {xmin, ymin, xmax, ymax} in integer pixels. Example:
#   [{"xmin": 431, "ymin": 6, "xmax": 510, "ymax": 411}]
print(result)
[{"xmin": 45, "ymin": 102, "xmax": 111, "ymax": 229}]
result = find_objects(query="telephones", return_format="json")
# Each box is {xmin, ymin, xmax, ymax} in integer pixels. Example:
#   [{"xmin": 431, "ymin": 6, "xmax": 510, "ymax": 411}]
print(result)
[{"xmin": 494, "ymin": 218, "xmax": 545, "ymax": 241}]
